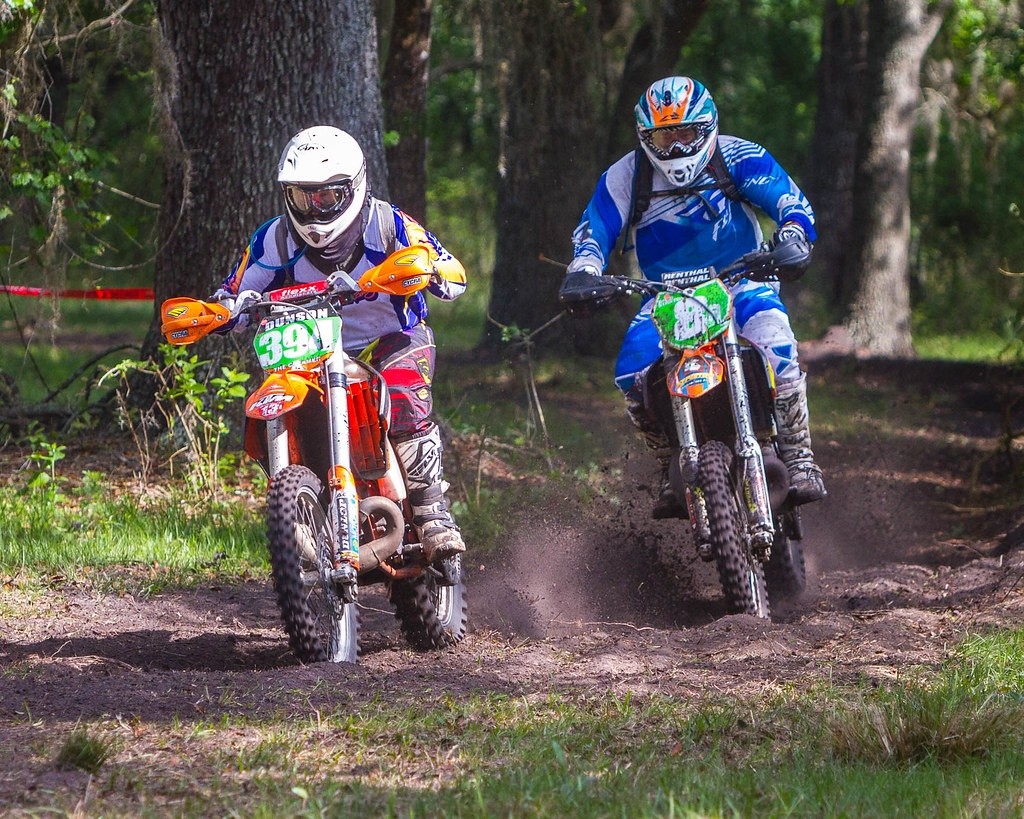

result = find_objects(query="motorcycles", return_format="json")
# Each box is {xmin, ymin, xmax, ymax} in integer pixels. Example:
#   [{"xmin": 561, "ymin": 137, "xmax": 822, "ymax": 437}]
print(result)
[
  {"xmin": 560, "ymin": 239, "xmax": 827, "ymax": 622},
  {"xmin": 160, "ymin": 244, "xmax": 469, "ymax": 663}
]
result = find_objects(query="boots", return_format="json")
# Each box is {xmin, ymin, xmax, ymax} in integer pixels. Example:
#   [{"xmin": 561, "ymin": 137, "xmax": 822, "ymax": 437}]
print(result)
[
  {"xmin": 772, "ymin": 370, "xmax": 827, "ymax": 503},
  {"xmin": 623, "ymin": 389, "xmax": 689, "ymax": 521},
  {"xmin": 390, "ymin": 422, "xmax": 466, "ymax": 563}
]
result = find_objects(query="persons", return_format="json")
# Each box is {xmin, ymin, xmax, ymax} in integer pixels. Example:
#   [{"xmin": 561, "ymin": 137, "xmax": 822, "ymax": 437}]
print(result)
[
  {"xmin": 199, "ymin": 125, "xmax": 467, "ymax": 564},
  {"xmin": 564, "ymin": 75, "xmax": 829, "ymax": 521}
]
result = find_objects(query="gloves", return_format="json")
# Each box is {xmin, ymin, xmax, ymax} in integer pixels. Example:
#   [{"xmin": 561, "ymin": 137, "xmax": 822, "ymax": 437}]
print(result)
[{"xmin": 773, "ymin": 221, "xmax": 806, "ymax": 245}]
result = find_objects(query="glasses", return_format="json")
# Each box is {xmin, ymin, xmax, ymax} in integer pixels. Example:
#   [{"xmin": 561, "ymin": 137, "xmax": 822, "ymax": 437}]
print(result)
[
  {"xmin": 636, "ymin": 105, "xmax": 719, "ymax": 161},
  {"xmin": 283, "ymin": 157, "xmax": 368, "ymax": 216}
]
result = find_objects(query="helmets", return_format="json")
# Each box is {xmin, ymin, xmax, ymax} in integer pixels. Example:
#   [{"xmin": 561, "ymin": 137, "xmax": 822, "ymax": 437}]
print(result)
[
  {"xmin": 277, "ymin": 126, "xmax": 372, "ymax": 265},
  {"xmin": 634, "ymin": 76, "xmax": 719, "ymax": 189}
]
